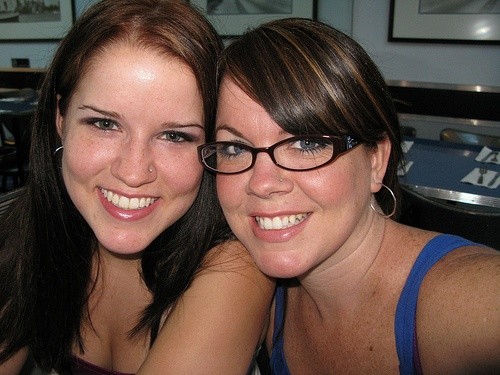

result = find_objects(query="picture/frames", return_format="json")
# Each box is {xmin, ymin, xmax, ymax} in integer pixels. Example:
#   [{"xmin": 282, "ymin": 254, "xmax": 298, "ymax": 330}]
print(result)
[
  {"xmin": 386, "ymin": 0, "xmax": 499, "ymax": 46},
  {"xmin": 185, "ymin": 0, "xmax": 319, "ymax": 40},
  {"xmin": 1, "ymin": 0, "xmax": 77, "ymax": 43}
]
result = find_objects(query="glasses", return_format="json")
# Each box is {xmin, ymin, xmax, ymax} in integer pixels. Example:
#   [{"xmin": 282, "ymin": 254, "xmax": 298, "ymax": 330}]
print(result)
[{"xmin": 197, "ymin": 135, "xmax": 364, "ymax": 175}]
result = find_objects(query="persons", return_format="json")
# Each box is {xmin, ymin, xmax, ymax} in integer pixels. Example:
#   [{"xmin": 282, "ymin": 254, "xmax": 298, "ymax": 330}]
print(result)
[
  {"xmin": 197, "ymin": 17, "xmax": 500, "ymax": 375},
  {"xmin": 0, "ymin": 1, "xmax": 275, "ymax": 375}
]
